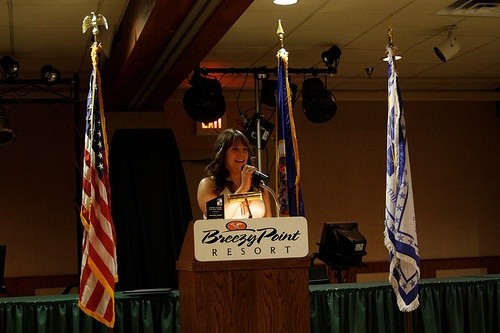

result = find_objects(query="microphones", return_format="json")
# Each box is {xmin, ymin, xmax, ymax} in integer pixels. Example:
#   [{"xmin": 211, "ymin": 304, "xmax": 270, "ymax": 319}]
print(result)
[{"xmin": 241, "ymin": 165, "xmax": 270, "ymax": 182}]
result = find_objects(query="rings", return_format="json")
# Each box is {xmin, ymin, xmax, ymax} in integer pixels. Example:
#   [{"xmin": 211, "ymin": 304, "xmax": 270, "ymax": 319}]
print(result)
[
  {"xmin": 245, "ymin": 171, "xmax": 248, "ymax": 174},
  {"xmin": 249, "ymin": 172, "xmax": 253, "ymax": 175},
  {"xmin": 242, "ymin": 169, "xmax": 244, "ymax": 171}
]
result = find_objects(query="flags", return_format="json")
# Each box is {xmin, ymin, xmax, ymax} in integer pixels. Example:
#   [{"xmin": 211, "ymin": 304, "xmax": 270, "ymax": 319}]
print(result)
[
  {"xmin": 383, "ymin": 57, "xmax": 420, "ymax": 312},
  {"xmin": 276, "ymin": 48, "xmax": 306, "ymax": 217},
  {"xmin": 77, "ymin": 42, "xmax": 119, "ymax": 329}
]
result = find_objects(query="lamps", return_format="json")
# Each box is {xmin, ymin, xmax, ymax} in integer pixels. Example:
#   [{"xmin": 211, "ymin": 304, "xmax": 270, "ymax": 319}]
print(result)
[
  {"xmin": 433, "ymin": 24, "xmax": 461, "ymax": 62},
  {"xmin": 0, "ymin": 54, "xmax": 60, "ymax": 86},
  {"xmin": 182, "ymin": 45, "xmax": 342, "ymax": 125}
]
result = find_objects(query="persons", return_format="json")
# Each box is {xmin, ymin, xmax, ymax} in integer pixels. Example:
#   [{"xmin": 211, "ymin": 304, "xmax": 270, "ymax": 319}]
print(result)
[{"xmin": 197, "ymin": 128, "xmax": 280, "ymax": 219}]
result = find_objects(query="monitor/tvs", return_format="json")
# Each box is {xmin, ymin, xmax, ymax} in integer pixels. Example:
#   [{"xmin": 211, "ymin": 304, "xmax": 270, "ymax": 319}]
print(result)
[{"xmin": 318, "ymin": 222, "xmax": 367, "ymax": 269}]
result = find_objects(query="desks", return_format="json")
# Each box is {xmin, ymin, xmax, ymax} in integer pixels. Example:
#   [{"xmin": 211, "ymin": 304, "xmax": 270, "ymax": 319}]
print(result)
[{"xmin": 0, "ymin": 274, "xmax": 500, "ymax": 333}]
